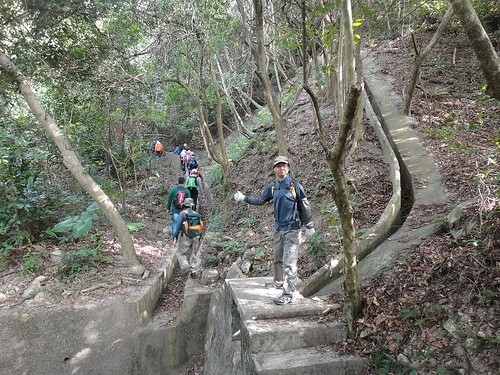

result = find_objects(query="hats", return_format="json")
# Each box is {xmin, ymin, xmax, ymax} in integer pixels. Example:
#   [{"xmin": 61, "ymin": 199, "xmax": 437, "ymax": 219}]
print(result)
[
  {"xmin": 191, "ymin": 169, "xmax": 197, "ymax": 173},
  {"xmin": 184, "ymin": 198, "xmax": 195, "ymax": 206},
  {"xmin": 274, "ymin": 156, "xmax": 289, "ymax": 165}
]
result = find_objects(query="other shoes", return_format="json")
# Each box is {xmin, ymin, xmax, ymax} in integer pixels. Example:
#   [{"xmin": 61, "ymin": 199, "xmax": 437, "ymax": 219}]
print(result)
[{"xmin": 182, "ymin": 268, "xmax": 198, "ymax": 279}]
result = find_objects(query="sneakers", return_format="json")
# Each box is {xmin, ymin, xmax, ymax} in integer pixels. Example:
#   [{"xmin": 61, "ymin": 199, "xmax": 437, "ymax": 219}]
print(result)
[
  {"xmin": 275, "ymin": 293, "xmax": 294, "ymax": 304},
  {"xmin": 265, "ymin": 282, "xmax": 283, "ymax": 289}
]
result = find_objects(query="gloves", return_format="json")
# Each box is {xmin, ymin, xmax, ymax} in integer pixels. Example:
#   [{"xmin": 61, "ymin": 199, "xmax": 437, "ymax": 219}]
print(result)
[
  {"xmin": 305, "ymin": 228, "xmax": 315, "ymax": 236},
  {"xmin": 234, "ymin": 191, "xmax": 245, "ymax": 202}
]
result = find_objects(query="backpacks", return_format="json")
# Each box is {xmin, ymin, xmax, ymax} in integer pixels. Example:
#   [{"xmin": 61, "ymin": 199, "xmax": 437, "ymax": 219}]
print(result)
[
  {"xmin": 189, "ymin": 157, "xmax": 195, "ymax": 164},
  {"xmin": 156, "ymin": 144, "xmax": 164, "ymax": 151},
  {"xmin": 182, "ymin": 210, "xmax": 203, "ymax": 239},
  {"xmin": 186, "ymin": 175, "xmax": 198, "ymax": 188},
  {"xmin": 174, "ymin": 187, "xmax": 186, "ymax": 210},
  {"xmin": 185, "ymin": 152, "xmax": 190, "ymax": 162}
]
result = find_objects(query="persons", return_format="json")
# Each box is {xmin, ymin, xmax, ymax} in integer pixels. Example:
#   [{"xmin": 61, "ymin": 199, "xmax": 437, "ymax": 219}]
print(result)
[
  {"xmin": 234, "ymin": 156, "xmax": 316, "ymax": 305},
  {"xmin": 167, "ymin": 143, "xmax": 203, "ymax": 243},
  {"xmin": 146, "ymin": 138, "xmax": 165, "ymax": 158},
  {"xmin": 172, "ymin": 198, "xmax": 203, "ymax": 278}
]
